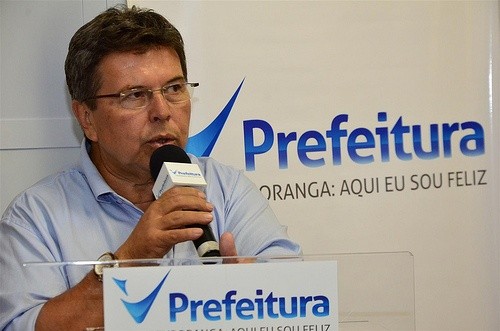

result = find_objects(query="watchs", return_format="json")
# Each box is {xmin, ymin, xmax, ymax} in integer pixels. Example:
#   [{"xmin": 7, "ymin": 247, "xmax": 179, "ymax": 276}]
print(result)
[{"xmin": 94, "ymin": 252, "xmax": 120, "ymax": 282}]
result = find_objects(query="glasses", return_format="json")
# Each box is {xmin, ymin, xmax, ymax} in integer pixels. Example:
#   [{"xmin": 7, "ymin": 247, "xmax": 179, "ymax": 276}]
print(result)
[{"xmin": 82, "ymin": 82, "xmax": 201, "ymax": 111}]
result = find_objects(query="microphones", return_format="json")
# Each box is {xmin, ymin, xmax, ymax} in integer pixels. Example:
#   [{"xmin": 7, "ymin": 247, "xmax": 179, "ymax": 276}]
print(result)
[{"xmin": 149, "ymin": 145, "xmax": 221, "ymax": 265}]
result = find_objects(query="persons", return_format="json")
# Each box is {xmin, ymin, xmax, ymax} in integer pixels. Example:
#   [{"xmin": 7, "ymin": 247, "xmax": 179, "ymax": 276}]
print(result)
[{"xmin": 0, "ymin": 3, "xmax": 305, "ymax": 331}]
